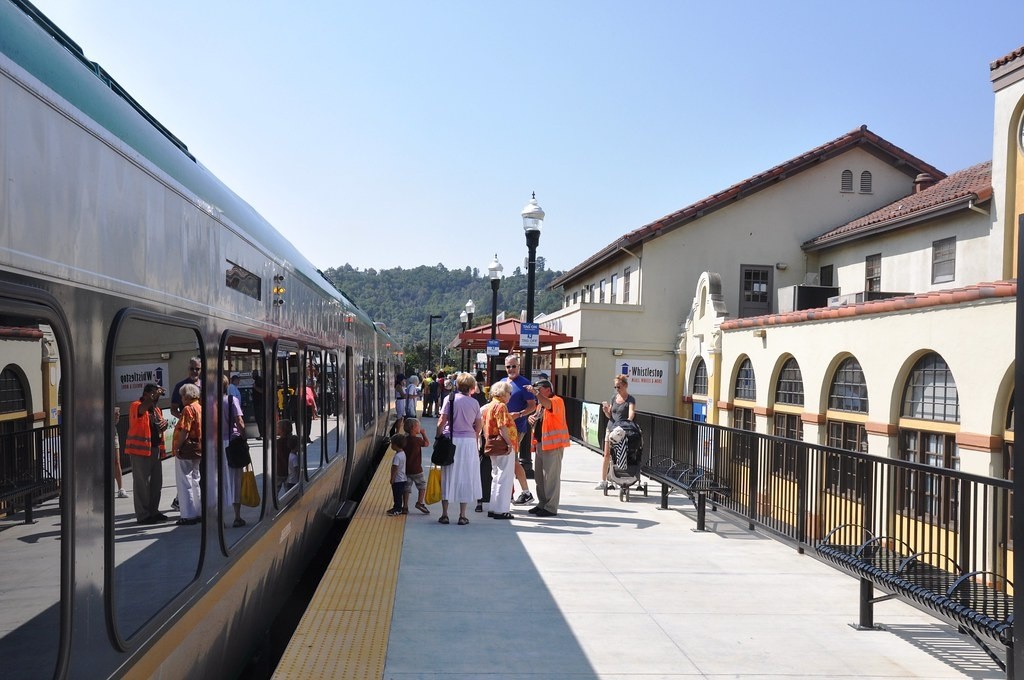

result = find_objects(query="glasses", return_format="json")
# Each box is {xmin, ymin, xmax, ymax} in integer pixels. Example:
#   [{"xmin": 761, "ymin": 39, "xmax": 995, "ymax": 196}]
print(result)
[
  {"xmin": 505, "ymin": 364, "xmax": 519, "ymax": 369},
  {"xmin": 504, "ymin": 391, "xmax": 512, "ymax": 396},
  {"xmin": 614, "ymin": 385, "xmax": 625, "ymax": 390},
  {"xmin": 188, "ymin": 367, "xmax": 200, "ymax": 372}
]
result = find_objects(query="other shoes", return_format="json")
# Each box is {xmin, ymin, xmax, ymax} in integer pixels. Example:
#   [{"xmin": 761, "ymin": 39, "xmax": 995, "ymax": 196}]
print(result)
[
  {"xmin": 387, "ymin": 506, "xmax": 402, "ymax": 516},
  {"xmin": 415, "ymin": 502, "xmax": 430, "ymax": 513},
  {"xmin": 422, "ymin": 411, "xmax": 442, "ymax": 418},
  {"xmin": 401, "ymin": 506, "xmax": 409, "ymax": 514},
  {"xmin": 118, "ymin": 487, "xmax": 247, "ymax": 530},
  {"xmin": 528, "ymin": 506, "xmax": 557, "ymax": 517},
  {"xmin": 475, "ymin": 504, "xmax": 482, "ymax": 512}
]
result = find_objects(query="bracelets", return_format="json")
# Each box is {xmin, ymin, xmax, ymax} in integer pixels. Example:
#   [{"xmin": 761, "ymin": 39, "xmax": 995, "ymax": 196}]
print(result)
[
  {"xmin": 519, "ymin": 412, "xmax": 523, "ymax": 418},
  {"xmin": 535, "ymin": 391, "xmax": 540, "ymax": 395}
]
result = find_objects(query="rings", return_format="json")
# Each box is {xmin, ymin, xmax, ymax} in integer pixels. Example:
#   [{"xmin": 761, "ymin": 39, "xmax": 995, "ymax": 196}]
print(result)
[{"xmin": 164, "ymin": 425, "xmax": 166, "ymax": 428}]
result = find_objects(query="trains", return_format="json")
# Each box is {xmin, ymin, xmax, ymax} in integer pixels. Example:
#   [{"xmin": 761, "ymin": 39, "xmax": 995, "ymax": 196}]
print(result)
[{"xmin": 1, "ymin": 0, "xmax": 403, "ymax": 680}]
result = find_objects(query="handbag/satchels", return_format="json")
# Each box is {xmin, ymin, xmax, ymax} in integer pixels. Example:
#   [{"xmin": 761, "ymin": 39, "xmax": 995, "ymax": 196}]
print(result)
[
  {"xmin": 424, "ymin": 464, "xmax": 442, "ymax": 506},
  {"xmin": 431, "ymin": 436, "xmax": 456, "ymax": 465},
  {"xmin": 175, "ymin": 440, "xmax": 202, "ymax": 460},
  {"xmin": 240, "ymin": 459, "xmax": 261, "ymax": 508},
  {"xmin": 225, "ymin": 436, "xmax": 251, "ymax": 468},
  {"xmin": 483, "ymin": 435, "xmax": 509, "ymax": 455}
]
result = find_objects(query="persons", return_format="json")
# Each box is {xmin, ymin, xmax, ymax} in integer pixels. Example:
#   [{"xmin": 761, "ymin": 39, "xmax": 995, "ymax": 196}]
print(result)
[
  {"xmin": 384, "ymin": 354, "xmax": 571, "ymax": 525},
  {"xmin": 114, "ymin": 357, "xmax": 381, "ymax": 527},
  {"xmin": 595, "ymin": 374, "xmax": 636, "ymax": 490}
]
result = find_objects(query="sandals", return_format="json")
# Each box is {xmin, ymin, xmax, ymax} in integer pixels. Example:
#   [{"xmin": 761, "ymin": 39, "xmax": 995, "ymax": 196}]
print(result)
[
  {"xmin": 438, "ymin": 515, "xmax": 449, "ymax": 525},
  {"xmin": 457, "ymin": 516, "xmax": 469, "ymax": 525}
]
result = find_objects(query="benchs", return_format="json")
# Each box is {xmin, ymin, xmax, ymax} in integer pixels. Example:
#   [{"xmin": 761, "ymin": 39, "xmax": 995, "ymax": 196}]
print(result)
[
  {"xmin": 640, "ymin": 455, "xmax": 732, "ymax": 532},
  {"xmin": 815, "ymin": 524, "xmax": 1014, "ymax": 680}
]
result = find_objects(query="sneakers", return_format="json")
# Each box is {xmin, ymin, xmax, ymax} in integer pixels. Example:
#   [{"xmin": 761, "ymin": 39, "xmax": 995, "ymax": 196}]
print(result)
[
  {"xmin": 513, "ymin": 492, "xmax": 532, "ymax": 506},
  {"xmin": 596, "ymin": 481, "xmax": 610, "ymax": 490}
]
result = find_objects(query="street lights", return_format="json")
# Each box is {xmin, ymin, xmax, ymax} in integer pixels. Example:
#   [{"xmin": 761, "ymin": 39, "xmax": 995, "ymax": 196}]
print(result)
[
  {"xmin": 428, "ymin": 314, "xmax": 441, "ymax": 368},
  {"xmin": 460, "ymin": 312, "xmax": 468, "ymax": 370},
  {"xmin": 488, "ymin": 253, "xmax": 503, "ymax": 386},
  {"xmin": 520, "ymin": 193, "xmax": 545, "ymax": 479},
  {"xmin": 466, "ymin": 300, "xmax": 476, "ymax": 373}
]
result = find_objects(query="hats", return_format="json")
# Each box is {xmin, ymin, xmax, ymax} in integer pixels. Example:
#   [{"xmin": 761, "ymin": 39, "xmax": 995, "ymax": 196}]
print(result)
[
  {"xmin": 143, "ymin": 383, "xmax": 162, "ymax": 392},
  {"xmin": 532, "ymin": 379, "xmax": 551, "ymax": 391},
  {"xmin": 538, "ymin": 373, "xmax": 548, "ymax": 379}
]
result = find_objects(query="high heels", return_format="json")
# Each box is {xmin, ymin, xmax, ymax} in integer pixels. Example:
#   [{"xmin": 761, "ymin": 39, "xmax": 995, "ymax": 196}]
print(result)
[{"xmin": 488, "ymin": 510, "xmax": 515, "ymax": 520}]
